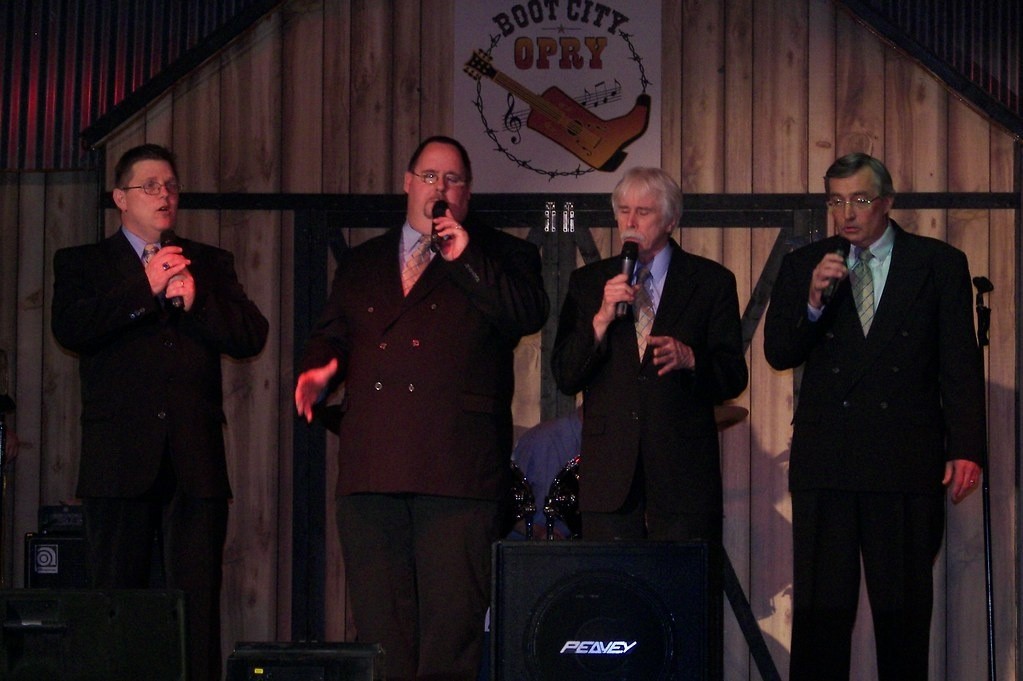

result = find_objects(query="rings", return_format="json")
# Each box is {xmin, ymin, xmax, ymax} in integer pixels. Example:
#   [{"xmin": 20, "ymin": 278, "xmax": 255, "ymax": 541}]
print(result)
[
  {"xmin": 970, "ymin": 480, "xmax": 974, "ymax": 483},
  {"xmin": 455, "ymin": 224, "xmax": 462, "ymax": 229},
  {"xmin": 162, "ymin": 261, "xmax": 170, "ymax": 270},
  {"xmin": 180, "ymin": 281, "xmax": 184, "ymax": 286}
]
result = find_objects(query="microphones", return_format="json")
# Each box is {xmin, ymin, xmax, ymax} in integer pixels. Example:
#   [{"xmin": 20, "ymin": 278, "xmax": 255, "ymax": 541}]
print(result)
[
  {"xmin": 820, "ymin": 236, "xmax": 851, "ymax": 305},
  {"xmin": 430, "ymin": 200, "xmax": 449, "ymax": 253},
  {"xmin": 159, "ymin": 228, "xmax": 185, "ymax": 310},
  {"xmin": 615, "ymin": 241, "xmax": 640, "ymax": 319}
]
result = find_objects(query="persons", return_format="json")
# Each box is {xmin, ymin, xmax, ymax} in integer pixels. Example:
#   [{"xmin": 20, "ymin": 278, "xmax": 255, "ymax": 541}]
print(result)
[
  {"xmin": 294, "ymin": 135, "xmax": 549, "ymax": 681},
  {"xmin": 48, "ymin": 143, "xmax": 268, "ymax": 681},
  {"xmin": 550, "ymin": 167, "xmax": 748, "ymax": 681},
  {"xmin": 763, "ymin": 152, "xmax": 988, "ymax": 681}
]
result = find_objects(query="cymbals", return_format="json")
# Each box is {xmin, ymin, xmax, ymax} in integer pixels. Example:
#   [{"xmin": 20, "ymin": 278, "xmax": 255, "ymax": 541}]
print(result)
[{"xmin": 713, "ymin": 403, "xmax": 749, "ymax": 429}]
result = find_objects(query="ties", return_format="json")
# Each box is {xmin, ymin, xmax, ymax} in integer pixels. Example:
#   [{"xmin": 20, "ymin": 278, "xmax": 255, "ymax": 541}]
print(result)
[
  {"xmin": 635, "ymin": 268, "xmax": 655, "ymax": 363},
  {"xmin": 851, "ymin": 249, "xmax": 875, "ymax": 338},
  {"xmin": 401, "ymin": 235, "xmax": 431, "ymax": 297},
  {"xmin": 144, "ymin": 244, "xmax": 159, "ymax": 266}
]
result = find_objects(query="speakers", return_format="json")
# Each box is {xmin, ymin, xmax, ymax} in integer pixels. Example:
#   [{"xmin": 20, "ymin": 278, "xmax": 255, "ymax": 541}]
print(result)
[
  {"xmin": 225, "ymin": 641, "xmax": 380, "ymax": 681},
  {"xmin": 1, "ymin": 530, "xmax": 188, "ymax": 681},
  {"xmin": 487, "ymin": 540, "xmax": 714, "ymax": 681}
]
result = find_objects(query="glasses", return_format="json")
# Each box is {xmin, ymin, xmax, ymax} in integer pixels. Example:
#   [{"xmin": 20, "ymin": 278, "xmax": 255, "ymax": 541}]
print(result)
[
  {"xmin": 411, "ymin": 172, "xmax": 467, "ymax": 188},
  {"xmin": 827, "ymin": 194, "xmax": 879, "ymax": 208},
  {"xmin": 121, "ymin": 182, "xmax": 184, "ymax": 195}
]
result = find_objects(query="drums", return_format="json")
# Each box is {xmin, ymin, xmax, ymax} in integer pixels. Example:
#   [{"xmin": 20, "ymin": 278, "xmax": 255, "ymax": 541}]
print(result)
[
  {"xmin": 547, "ymin": 457, "xmax": 583, "ymax": 535},
  {"xmin": 504, "ymin": 461, "xmax": 538, "ymax": 538}
]
